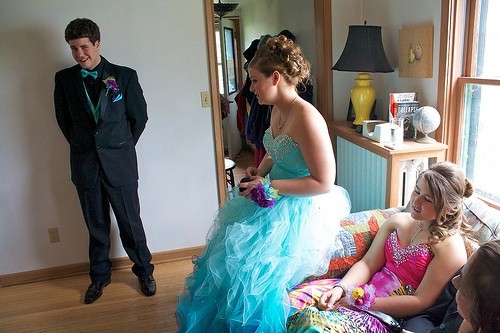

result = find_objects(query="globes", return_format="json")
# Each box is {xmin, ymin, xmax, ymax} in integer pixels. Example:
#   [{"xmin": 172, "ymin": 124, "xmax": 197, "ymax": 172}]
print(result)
[{"xmin": 412, "ymin": 105, "xmax": 442, "ymax": 143}]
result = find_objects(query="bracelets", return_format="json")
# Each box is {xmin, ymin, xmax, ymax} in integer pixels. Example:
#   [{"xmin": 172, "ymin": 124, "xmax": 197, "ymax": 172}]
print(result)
[{"xmin": 333, "ymin": 284, "xmax": 347, "ymax": 297}]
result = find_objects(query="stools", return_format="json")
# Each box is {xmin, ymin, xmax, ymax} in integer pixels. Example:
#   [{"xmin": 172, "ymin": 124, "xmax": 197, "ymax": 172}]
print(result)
[{"xmin": 225, "ymin": 158, "xmax": 236, "ymax": 189}]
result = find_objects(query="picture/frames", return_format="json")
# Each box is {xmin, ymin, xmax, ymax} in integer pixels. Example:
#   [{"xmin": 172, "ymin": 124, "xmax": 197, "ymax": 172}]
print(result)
[{"xmin": 347, "ymin": 97, "xmax": 376, "ymax": 121}]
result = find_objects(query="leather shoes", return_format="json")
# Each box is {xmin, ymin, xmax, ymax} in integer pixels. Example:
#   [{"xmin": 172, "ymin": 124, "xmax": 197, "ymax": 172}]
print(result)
[
  {"xmin": 138, "ymin": 275, "xmax": 157, "ymax": 296},
  {"xmin": 84, "ymin": 279, "xmax": 111, "ymax": 303}
]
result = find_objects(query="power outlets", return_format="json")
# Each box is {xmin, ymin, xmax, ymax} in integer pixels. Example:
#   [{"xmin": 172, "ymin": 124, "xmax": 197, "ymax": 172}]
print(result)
[
  {"xmin": 48, "ymin": 228, "xmax": 60, "ymax": 242},
  {"xmin": 200, "ymin": 91, "xmax": 211, "ymax": 106}
]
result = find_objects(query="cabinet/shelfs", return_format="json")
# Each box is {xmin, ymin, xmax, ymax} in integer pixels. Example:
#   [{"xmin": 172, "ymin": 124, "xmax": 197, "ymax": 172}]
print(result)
[{"xmin": 333, "ymin": 119, "xmax": 448, "ymax": 214}]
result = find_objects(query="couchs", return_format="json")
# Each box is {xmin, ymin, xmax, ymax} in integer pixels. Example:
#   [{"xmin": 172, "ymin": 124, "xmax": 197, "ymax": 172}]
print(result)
[{"xmin": 304, "ymin": 197, "xmax": 500, "ymax": 284}]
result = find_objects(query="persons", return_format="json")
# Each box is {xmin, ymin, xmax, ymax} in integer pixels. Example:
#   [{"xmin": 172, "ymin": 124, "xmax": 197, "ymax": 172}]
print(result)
[
  {"xmin": 175, "ymin": 33, "xmax": 352, "ymax": 333},
  {"xmin": 54, "ymin": 19, "xmax": 156, "ymax": 305},
  {"xmin": 451, "ymin": 239, "xmax": 500, "ymax": 333},
  {"xmin": 285, "ymin": 161, "xmax": 472, "ymax": 333}
]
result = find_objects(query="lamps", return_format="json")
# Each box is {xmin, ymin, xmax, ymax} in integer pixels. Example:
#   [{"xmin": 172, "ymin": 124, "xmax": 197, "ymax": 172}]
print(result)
[
  {"xmin": 330, "ymin": 20, "xmax": 396, "ymax": 126},
  {"xmin": 214, "ymin": 0, "xmax": 239, "ymax": 20}
]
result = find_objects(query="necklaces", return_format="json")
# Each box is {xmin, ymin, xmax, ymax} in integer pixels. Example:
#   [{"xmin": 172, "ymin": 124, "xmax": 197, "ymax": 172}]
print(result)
[
  {"xmin": 411, "ymin": 219, "xmax": 428, "ymax": 243},
  {"xmin": 277, "ymin": 95, "xmax": 298, "ymax": 131}
]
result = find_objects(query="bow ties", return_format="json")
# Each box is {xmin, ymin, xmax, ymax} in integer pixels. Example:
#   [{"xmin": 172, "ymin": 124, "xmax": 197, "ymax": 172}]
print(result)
[{"xmin": 81, "ymin": 69, "xmax": 98, "ymax": 80}]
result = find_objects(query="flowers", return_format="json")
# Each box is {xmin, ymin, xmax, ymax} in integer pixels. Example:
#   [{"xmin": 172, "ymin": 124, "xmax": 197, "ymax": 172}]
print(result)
[{"xmin": 102, "ymin": 76, "xmax": 118, "ymax": 96}]
